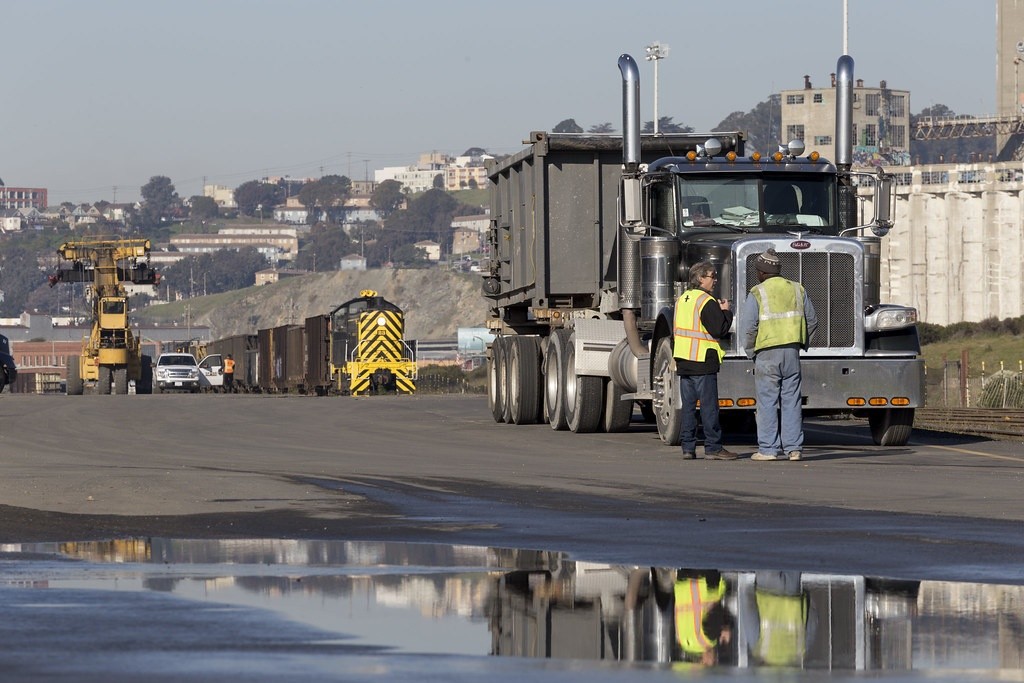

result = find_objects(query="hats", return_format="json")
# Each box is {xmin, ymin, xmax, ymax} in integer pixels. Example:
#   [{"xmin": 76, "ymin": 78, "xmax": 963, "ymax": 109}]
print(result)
[{"xmin": 755, "ymin": 248, "xmax": 780, "ymax": 274}]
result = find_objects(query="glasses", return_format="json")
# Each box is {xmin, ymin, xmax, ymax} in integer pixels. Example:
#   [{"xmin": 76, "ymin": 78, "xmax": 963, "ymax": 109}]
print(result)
[{"xmin": 706, "ymin": 274, "xmax": 717, "ymax": 279}]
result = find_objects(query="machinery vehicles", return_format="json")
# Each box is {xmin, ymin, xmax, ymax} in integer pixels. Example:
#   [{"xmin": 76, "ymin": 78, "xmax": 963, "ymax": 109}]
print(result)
[{"xmin": 48, "ymin": 234, "xmax": 162, "ymax": 395}]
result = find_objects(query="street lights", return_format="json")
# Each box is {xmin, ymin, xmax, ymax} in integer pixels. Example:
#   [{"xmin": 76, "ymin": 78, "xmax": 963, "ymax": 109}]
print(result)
[{"xmin": 645, "ymin": 45, "xmax": 665, "ymax": 134}]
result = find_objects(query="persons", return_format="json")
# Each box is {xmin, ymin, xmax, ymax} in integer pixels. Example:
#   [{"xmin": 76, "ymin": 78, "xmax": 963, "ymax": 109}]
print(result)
[
  {"xmin": 671, "ymin": 567, "xmax": 734, "ymax": 673},
  {"xmin": 218, "ymin": 354, "xmax": 236, "ymax": 394},
  {"xmin": 741, "ymin": 245, "xmax": 818, "ymax": 461},
  {"xmin": 738, "ymin": 572, "xmax": 819, "ymax": 670},
  {"xmin": 671, "ymin": 258, "xmax": 739, "ymax": 461}
]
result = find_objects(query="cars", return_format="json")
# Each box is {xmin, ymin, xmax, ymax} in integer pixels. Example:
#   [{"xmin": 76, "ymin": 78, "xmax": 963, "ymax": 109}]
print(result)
[{"xmin": 154, "ymin": 353, "xmax": 224, "ymax": 393}]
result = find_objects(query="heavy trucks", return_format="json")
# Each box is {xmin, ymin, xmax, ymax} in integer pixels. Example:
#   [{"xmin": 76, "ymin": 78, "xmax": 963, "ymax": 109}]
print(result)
[{"xmin": 482, "ymin": 54, "xmax": 924, "ymax": 446}]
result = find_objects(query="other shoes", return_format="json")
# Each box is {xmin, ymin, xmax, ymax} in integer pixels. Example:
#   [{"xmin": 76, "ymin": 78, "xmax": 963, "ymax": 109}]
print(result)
[
  {"xmin": 683, "ymin": 451, "xmax": 696, "ymax": 459},
  {"xmin": 751, "ymin": 452, "xmax": 776, "ymax": 461},
  {"xmin": 705, "ymin": 448, "xmax": 737, "ymax": 460},
  {"xmin": 788, "ymin": 451, "xmax": 801, "ymax": 460}
]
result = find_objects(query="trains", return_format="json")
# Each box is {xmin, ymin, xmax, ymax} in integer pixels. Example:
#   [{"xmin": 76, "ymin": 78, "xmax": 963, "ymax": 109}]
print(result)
[{"xmin": 168, "ymin": 289, "xmax": 419, "ymax": 396}]
